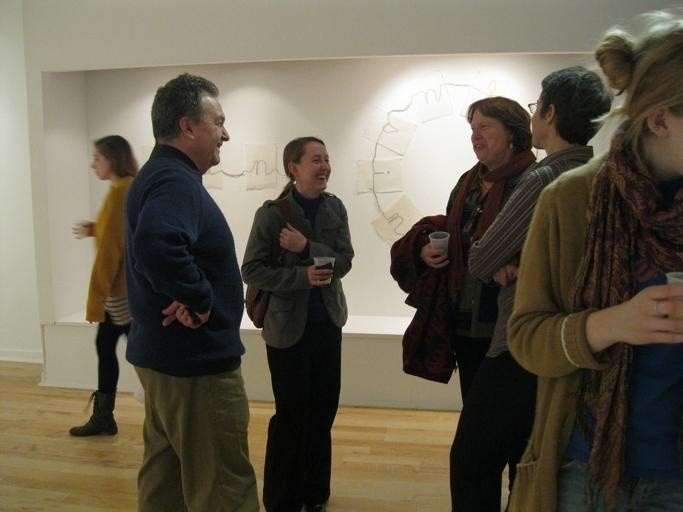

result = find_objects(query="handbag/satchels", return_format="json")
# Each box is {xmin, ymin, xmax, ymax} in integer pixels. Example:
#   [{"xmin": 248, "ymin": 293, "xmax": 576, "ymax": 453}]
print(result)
[
  {"xmin": 401, "ymin": 309, "xmax": 455, "ymax": 384},
  {"xmin": 245, "ymin": 261, "xmax": 280, "ymax": 328}
]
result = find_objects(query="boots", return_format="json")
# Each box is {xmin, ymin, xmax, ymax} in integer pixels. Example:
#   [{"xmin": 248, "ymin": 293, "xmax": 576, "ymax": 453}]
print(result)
[{"xmin": 69, "ymin": 390, "xmax": 115, "ymax": 437}]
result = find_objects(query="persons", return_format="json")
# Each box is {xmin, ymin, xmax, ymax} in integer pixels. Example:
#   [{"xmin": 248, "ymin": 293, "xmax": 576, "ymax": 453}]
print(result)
[
  {"xmin": 505, "ymin": 7, "xmax": 681, "ymax": 510},
  {"xmin": 239, "ymin": 138, "xmax": 357, "ymax": 511},
  {"xmin": 120, "ymin": 74, "xmax": 264, "ymax": 511},
  {"xmin": 68, "ymin": 135, "xmax": 141, "ymax": 438},
  {"xmin": 424, "ymin": 95, "xmax": 538, "ymax": 410},
  {"xmin": 442, "ymin": 65, "xmax": 612, "ymax": 512}
]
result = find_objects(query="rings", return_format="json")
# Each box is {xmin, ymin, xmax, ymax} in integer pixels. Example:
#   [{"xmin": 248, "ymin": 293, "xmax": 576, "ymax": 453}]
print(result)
[{"xmin": 652, "ymin": 298, "xmax": 662, "ymax": 318}]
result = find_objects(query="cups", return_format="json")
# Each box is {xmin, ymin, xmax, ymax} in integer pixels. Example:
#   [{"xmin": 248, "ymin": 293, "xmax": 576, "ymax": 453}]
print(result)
[
  {"xmin": 665, "ymin": 271, "xmax": 683, "ymax": 321},
  {"xmin": 314, "ymin": 255, "xmax": 336, "ymax": 285},
  {"xmin": 428, "ymin": 231, "xmax": 450, "ymax": 259}
]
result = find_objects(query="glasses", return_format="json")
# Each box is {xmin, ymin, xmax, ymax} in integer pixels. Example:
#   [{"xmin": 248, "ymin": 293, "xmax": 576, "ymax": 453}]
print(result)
[{"xmin": 528, "ymin": 102, "xmax": 549, "ymax": 115}]
